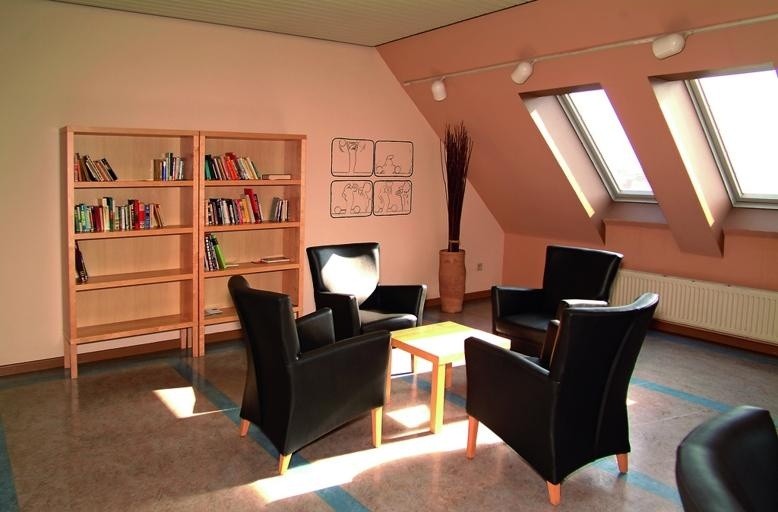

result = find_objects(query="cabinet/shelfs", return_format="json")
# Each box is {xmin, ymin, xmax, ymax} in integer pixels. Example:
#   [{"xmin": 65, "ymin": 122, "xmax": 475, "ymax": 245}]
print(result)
[
  {"xmin": 57, "ymin": 124, "xmax": 200, "ymax": 380},
  {"xmin": 194, "ymin": 129, "xmax": 309, "ymax": 359}
]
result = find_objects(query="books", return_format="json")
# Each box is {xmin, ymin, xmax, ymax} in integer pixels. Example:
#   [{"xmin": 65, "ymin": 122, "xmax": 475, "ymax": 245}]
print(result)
[{"xmin": 74, "ymin": 150, "xmax": 294, "ymax": 283}]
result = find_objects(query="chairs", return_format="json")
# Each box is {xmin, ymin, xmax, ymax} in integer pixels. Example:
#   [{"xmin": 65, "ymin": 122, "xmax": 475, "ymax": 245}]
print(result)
[
  {"xmin": 462, "ymin": 288, "xmax": 660, "ymax": 506},
  {"xmin": 222, "ymin": 264, "xmax": 384, "ymax": 478},
  {"xmin": 487, "ymin": 242, "xmax": 627, "ymax": 362},
  {"xmin": 672, "ymin": 402, "xmax": 776, "ymax": 512},
  {"xmin": 304, "ymin": 237, "xmax": 430, "ymax": 376}
]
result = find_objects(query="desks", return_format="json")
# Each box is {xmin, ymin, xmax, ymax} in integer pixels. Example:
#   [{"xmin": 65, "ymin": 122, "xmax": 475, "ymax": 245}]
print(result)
[{"xmin": 381, "ymin": 319, "xmax": 513, "ymax": 440}]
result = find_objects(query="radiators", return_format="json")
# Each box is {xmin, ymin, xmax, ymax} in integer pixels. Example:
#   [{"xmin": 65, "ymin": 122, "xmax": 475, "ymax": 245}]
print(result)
[{"xmin": 607, "ymin": 266, "xmax": 778, "ymax": 351}]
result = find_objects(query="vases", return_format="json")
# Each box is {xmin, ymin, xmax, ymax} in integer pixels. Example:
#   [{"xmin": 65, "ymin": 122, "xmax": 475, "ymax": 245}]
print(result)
[{"xmin": 436, "ymin": 246, "xmax": 468, "ymax": 314}]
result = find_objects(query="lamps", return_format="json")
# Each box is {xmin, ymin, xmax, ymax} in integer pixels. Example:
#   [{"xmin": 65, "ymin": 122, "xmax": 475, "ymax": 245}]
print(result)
[{"xmin": 429, "ymin": 28, "xmax": 695, "ymax": 101}]
